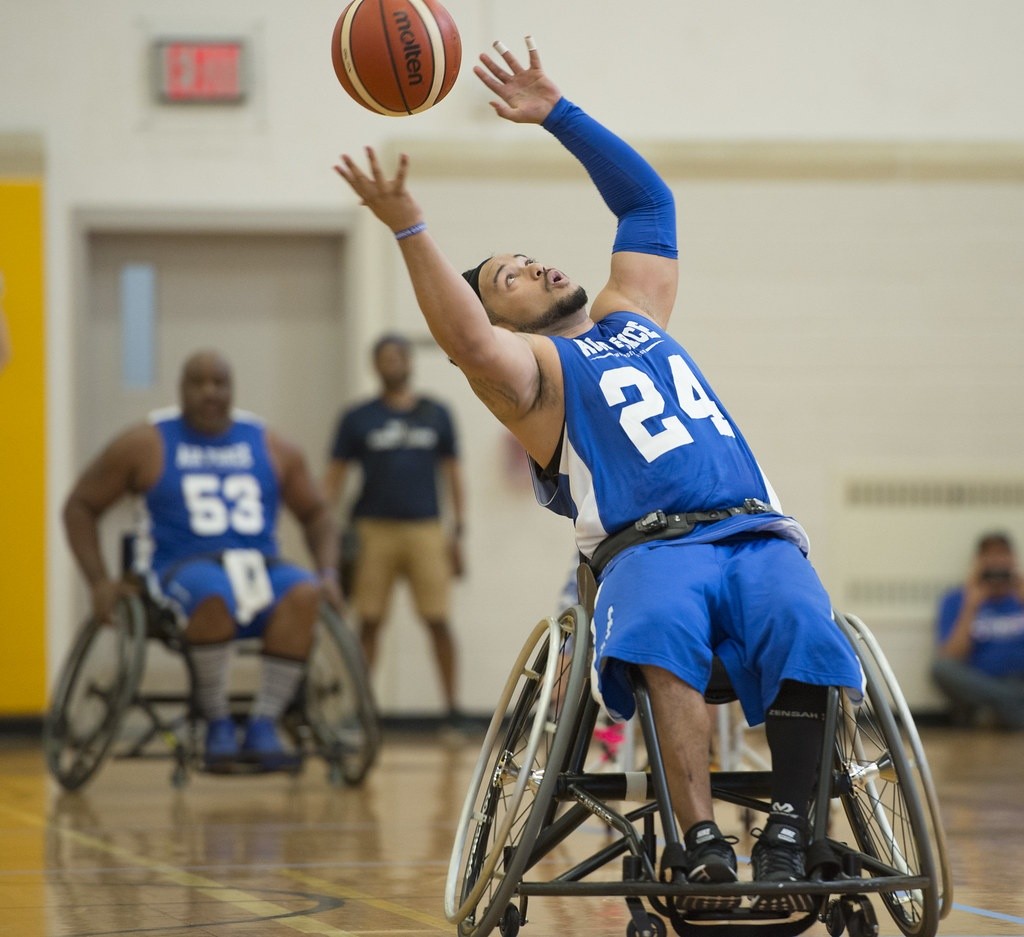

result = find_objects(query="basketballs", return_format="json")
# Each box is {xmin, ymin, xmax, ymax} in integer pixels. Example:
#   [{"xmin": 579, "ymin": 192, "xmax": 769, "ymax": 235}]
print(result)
[{"xmin": 331, "ymin": 0, "xmax": 461, "ymax": 118}]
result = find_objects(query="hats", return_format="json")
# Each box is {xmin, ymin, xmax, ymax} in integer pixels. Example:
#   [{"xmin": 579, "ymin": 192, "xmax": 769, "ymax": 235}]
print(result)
[{"xmin": 449, "ymin": 255, "xmax": 492, "ymax": 369}]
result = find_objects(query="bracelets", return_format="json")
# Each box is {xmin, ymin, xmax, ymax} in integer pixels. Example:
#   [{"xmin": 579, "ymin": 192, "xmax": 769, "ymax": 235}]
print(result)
[{"xmin": 393, "ymin": 222, "xmax": 425, "ymax": 243}]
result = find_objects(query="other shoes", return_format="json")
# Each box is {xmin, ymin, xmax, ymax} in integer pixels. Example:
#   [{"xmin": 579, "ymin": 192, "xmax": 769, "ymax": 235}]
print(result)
[
  {"xmin": 203, "ymin": 718, "xmax": 239, "ymax": 773},
  {"xmin": 239, "ymin": 716, "xmax": 302, "ymax": 769}
]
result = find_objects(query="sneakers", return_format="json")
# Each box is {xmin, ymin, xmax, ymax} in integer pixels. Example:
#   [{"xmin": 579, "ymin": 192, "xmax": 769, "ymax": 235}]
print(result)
[
  {"xmin": 747, "ymin": 822, "xmax": 815, "ymax": 913},
  {"xmin": 673, "ymin": 824, "xmax": 743, "ymax": 911}
]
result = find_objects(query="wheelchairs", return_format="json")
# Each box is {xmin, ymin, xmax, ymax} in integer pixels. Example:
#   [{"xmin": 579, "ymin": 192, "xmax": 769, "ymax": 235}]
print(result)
[
  {"xmin": 41, "ymin": 531, "xmax": 380, "ymax": 795},
  {"xmin": 442, "ymin": 498, "xmax": 953, "ymax": 937}
]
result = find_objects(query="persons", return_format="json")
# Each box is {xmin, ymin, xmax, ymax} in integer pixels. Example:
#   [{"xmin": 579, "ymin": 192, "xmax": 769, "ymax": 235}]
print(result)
[
  {"xmin": 330, "ymin": 34, "xmax": 865, "ymax": 916},
  {"xmin": 925, "ymin": 535, "xmax": 1024, "ymax": 739},
  {"xmin": 317, "ymin": 340, "xmax": 481, "ymax": 743},
  {"xmin": 60, "ymin": 350, "xmax": 347, "ymax": 767}
]
